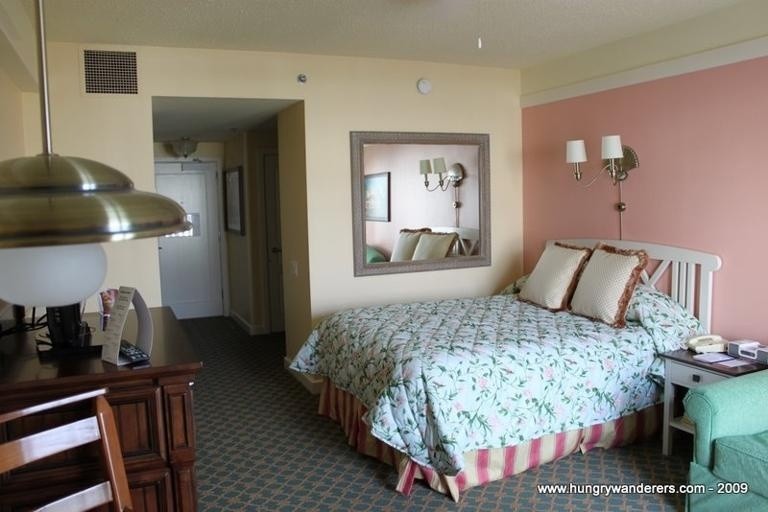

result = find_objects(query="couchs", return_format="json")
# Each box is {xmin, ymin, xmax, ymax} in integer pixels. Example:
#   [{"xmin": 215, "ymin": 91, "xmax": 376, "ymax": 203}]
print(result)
[{"xmin": 681, "ymin": 369, "xmax": 768, "ymax": 511}]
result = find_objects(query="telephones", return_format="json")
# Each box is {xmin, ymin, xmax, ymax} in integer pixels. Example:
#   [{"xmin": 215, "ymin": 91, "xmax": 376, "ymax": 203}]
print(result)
[{"xmin": 687, "ymin": 334, "xmax": 729, "ymax": 353}]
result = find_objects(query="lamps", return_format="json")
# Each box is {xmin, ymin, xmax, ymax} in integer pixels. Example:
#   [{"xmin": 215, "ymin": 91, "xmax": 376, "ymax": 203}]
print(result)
[
  {"xmin": 2, "ymin": 1, "xmax": 196, "ymax": 335},
  {"xmin": 419, "ymin": 157, "xmax": 465, "ymax": 228},
  {"xmin": 564, "ymin": 133, "xmax": 640, "ymax": 239},
  {"xmin": 168, "ymin": 138, "xmax": 199, "ymax": 158}
]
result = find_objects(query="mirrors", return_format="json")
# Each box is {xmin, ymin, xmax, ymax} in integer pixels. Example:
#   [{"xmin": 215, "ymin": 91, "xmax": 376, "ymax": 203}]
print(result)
[{"xmin": 349, "ymin": 130, "xmax": 493, "ymax": 278}]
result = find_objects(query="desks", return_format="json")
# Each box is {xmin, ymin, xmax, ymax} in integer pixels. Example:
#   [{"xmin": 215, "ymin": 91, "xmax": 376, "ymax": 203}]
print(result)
[{"xmin": 1, "ymin": 305, "xmax": 204, "ymax": 512}]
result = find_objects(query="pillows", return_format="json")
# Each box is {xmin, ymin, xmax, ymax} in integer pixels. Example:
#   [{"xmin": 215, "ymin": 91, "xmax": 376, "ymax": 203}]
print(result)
[
  {"xmin": 390, "ymin": 228, "xmax": 431, "ymax": 262},
  {"xmin": 567, "ymin": 242, "xmax": 648, "ymax": 328},
  {"xmin": 412, "ymin": 232, "xmax": 458, "ymax": 260},
  {"xmin": 517, "ymin": 244, "xmax": 593, "ymax": 313}
]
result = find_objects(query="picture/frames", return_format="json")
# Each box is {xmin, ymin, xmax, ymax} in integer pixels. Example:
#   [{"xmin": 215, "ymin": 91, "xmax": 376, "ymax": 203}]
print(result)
[
  {"xmin": 223, "ymin": 167, "xmax": 244, "ymax": 234},
  {"xmin": 362, "ymin": 173, "xmax": 389, "ymax": 222}
]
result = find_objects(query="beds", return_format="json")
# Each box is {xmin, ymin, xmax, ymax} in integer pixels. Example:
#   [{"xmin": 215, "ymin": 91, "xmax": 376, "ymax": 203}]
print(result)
[
  {"xmin": 288, "ymin": 239, "xmax": 722, "ymax": 504},
  {"xmin": 657, "ymin": 333, "xmax": 764, "ymax": 457}
]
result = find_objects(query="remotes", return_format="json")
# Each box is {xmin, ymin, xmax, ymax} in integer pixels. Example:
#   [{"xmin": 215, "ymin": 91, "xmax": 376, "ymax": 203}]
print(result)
[{"xmin": 120, "ymin": 340, "xmax": 150, "ymax": 362}]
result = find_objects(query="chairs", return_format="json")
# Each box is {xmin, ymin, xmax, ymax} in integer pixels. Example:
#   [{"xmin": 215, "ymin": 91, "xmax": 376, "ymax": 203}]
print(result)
[{"xmin": 1, "ymin": 386, "xmax": 132, "ymax": 511}]
